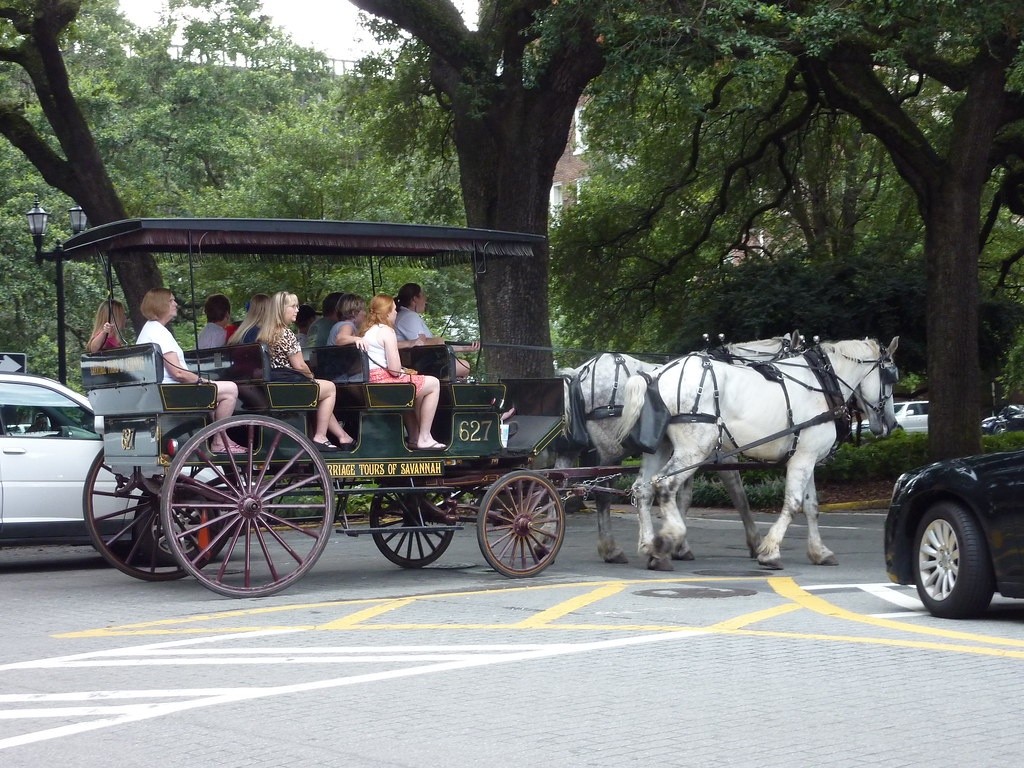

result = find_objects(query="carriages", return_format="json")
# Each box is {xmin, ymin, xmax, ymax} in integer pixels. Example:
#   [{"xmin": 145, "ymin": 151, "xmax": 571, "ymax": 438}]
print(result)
[{"xmin": 60, "ymin": 217, "xmax": 902, "ymax": 599}]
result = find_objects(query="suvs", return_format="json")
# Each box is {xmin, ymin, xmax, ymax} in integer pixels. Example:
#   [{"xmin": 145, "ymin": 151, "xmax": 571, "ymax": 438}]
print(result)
[{"xmin": 0, "ymin": 371, "xmax": 259, "ymax": 568}]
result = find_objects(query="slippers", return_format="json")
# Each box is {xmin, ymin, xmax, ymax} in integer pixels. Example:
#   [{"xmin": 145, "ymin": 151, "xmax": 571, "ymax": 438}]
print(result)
[
  {"xmin": 212, "ymin": 443, "xmax": 248, "ymax": 454},
  {"xmin": 420, "ymin": 442, "xmax": 446, "ymax": 450}
]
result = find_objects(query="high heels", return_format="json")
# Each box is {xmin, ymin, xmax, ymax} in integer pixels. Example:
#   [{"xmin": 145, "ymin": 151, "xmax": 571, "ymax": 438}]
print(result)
[
  {"xmin": 339, "ymin": 439, "xmax": 358, "ymax": 451},
  {"xmin": 314, "ymin": 439, "xmax": 340, "ymax": 451}
]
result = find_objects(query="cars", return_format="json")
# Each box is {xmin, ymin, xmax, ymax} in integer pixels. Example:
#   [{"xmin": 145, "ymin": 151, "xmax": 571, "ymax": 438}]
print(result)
[
  {"xmin": 882, "ymin": 448, "xmax": 1024, "ymax": 619},
  {"xmin": 980, "ymin": 405, "xmax": 1024, "ymax": 436},
  {"xmin": 848, "ymin": 400, "xmax": 930, "ymax": 435}
]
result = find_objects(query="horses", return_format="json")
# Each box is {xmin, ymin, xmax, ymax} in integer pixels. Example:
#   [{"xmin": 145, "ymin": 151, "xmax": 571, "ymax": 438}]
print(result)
[
  {"xmin": 534, "ymin": 330, "xmax": 803, "ymax": 564},
  {"xmin": 620, "ymin": 335, "xmax": 900, "ymax": 572}
]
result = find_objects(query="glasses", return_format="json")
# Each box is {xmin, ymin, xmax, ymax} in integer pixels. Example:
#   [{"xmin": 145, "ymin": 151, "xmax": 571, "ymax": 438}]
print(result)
[{"xmin": 285, "ymin": 304, "xmax": 300, "ymax": 310}]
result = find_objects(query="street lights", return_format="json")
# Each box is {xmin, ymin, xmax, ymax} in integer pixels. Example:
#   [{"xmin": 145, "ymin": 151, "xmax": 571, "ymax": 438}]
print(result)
[{"xmin": 24, "ymin": 194, "xmax": 88, "ymax": 386}]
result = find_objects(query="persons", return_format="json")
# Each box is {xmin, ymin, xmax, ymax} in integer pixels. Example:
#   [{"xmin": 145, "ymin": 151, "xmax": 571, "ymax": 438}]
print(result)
[
  {"xmin": 255, "ymin": 292, "xmax": 358, "ymax": 452},
  {"xmin": 357, "ymin": 294, "xmax": 446, "ymax": 451},
  {"xmin": 306, "ymin": 292, "xmax": 344, "ymax": 346},
  {"xmin": 136, "ymin": 286, "xmax": 248, "ymax": 455},
  {"xmin": 326, "ymin": 292, "xmax": 410, "ymax": 448},
  {"xmin": 294, "ymin": 304, "xmax": 317, "ymax": 360},
  {"xmin": 227, "ymin": 293, "xmax": 269, "ymax": 379},
  {"xmin": 86, "ymin": 300, "xmax": 126, "ymax": 353},
  {"xmin": 196, "ymin": 291, "xmax": 237, "ymax": 349},
  {"xmin": 393, "ymin": 282, "xmax": 516, "ymax": 423}
]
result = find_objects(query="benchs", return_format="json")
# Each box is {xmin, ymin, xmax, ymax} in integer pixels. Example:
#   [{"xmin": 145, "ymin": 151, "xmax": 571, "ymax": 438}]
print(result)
[
  {"xmin": 395, "ymin": 348, "xmax": 508, "ymax": 455},
  {"xmin": 185, "ymin": 340, "xmax": 322, "ymax": 454},
  {"xmin": 77, "ymin": 344, "xmax": 217, "ymax": 467},
  {"xmin": 300, "ymin": 345, "xmax": 417, "ymax": 458}
]
